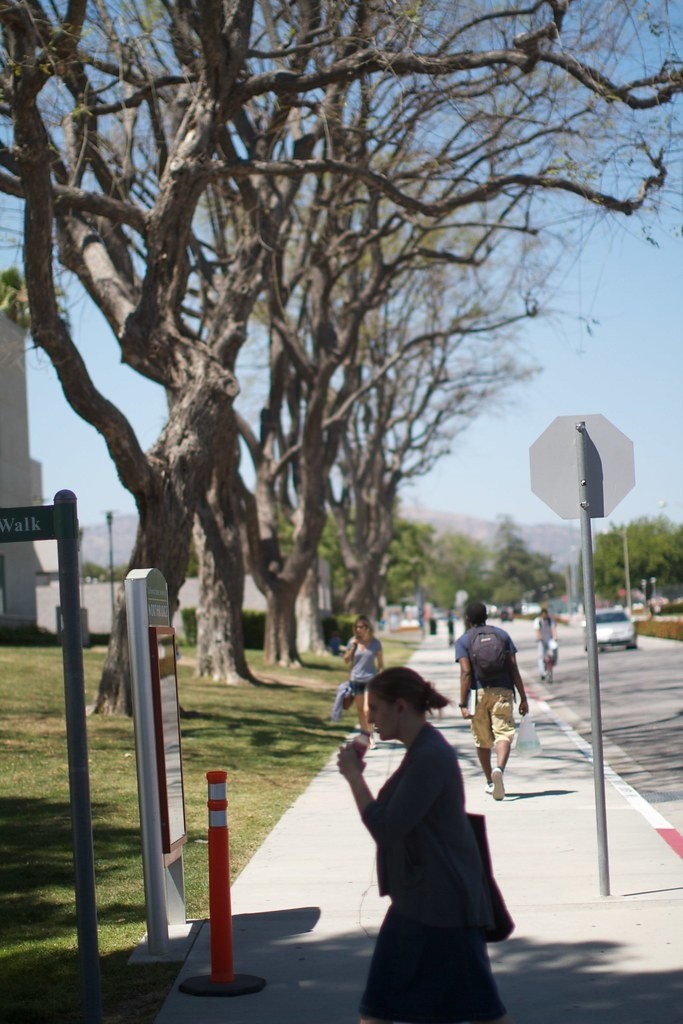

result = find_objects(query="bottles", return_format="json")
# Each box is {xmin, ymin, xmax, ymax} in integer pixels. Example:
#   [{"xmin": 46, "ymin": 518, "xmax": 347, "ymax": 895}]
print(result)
[{"xmin": 352, "ymin": 730, "xmax": 371, "ymax": 760}]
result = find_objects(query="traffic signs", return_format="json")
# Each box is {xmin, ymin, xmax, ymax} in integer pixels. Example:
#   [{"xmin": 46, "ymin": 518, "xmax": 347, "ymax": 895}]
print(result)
[{"xmin": 0, "ymin": 503, "xmax": 54, "ymax": 543}]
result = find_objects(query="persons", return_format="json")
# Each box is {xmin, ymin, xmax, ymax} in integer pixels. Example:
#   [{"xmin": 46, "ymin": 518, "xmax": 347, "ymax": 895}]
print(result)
[
  {"xmin": 344, "ymin": 615, "xmax": 384, "ymax": 750},
  {"xmin": 453, "ymin": 601, "xmax": 529, "ymax": 800},
  {"xmin": 337, "ymin": 669, "xmax": 517, "ymax": 1024},
  {"xmin": 534, "ymin": 609, "xmax": 559, "ymax": 680}
]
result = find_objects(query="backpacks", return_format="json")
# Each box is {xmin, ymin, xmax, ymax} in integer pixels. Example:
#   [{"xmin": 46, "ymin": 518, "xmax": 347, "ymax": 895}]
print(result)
[{"xmin": 464, "ymin": 626, "xmax": 518, "ymax": 704}]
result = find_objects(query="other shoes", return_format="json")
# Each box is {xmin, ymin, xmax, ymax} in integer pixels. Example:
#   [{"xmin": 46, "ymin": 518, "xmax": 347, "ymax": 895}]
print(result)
[
  {"xmin": 489, "ymin": 766, "xmax": 506, "ymax": 801},
  {"xmin": 485, "ymin": 783, "xmax": 494, "ymax": 794}
]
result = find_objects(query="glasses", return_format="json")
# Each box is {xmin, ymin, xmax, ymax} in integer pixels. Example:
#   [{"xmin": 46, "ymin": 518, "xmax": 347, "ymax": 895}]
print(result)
[{"xmin": 355, "ymin": 623, "xmax": 366, "ymax": 629}]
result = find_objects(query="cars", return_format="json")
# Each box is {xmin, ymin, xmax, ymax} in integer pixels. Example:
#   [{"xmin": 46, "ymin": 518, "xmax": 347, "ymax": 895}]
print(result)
[{"xmin": 582, "ymin": 610, "xmax": 635, "ymax": 652}]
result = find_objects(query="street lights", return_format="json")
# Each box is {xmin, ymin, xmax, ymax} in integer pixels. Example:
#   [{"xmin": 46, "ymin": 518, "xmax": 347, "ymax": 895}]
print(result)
[
  {"xmin": 106, "ymin": 512, "xmax": 114, "ymax": 616},
  {"xmin": 623, "ymin": 500, "xmax": 666, "ymax": 614}
]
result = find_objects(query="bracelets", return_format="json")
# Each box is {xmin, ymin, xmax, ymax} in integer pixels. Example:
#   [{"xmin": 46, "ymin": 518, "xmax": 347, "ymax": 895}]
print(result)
[{"xmin": 458, "ymin": 703, "xmax": 467, "ymax": 707}]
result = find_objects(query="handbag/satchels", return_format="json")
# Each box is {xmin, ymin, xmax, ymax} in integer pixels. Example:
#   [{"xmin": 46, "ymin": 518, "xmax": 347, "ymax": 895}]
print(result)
[
  {"xmin": 342, "ymin": 694, "xmax": 354, "ymax": 709},
  {"xmin": 466, "ymin": 812, "xmax": 516, "ymax": 943},
  {"xmin": 514, "ymin": 712, "xmax": 542, "ymax": 757}
]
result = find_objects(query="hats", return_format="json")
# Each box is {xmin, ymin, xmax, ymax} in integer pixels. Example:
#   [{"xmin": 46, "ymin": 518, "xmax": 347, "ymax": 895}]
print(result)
[{"xmin": 464, "ymin": 602, "xmax": 488, "ymax": 624}]
